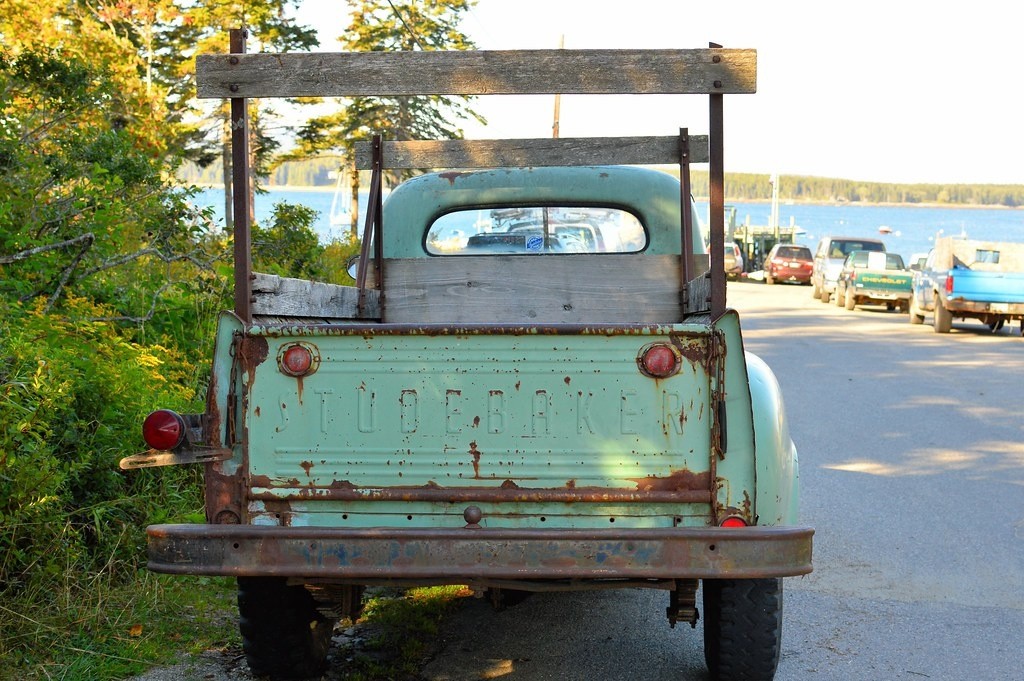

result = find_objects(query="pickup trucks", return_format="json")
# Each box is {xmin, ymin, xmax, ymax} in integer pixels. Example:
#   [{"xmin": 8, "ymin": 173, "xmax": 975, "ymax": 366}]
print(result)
[
  {"xmin": 910, "ymin": 236, "xmax": 1024, "ymax": 335},
  {"xmin": 834, "ymin": 250, "xmax": 915, "ymax": 315}
]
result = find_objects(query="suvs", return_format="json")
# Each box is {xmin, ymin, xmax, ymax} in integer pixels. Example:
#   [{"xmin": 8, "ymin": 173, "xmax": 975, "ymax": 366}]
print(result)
[{"xmin": 811, "ymin": 236, "xmax": 887, "ymax": 302}]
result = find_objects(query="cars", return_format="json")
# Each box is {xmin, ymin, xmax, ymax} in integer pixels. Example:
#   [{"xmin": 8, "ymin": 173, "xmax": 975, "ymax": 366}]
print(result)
[
  {"xmin": 706, "ymin": 240, "xmax": 743, "ymax": 279},
  {"xmin": 762, "ymin": 242, "xmax": 814, "ymax": 286}
]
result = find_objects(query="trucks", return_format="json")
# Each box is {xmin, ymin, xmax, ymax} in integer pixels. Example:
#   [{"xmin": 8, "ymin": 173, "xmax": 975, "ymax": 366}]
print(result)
[{"xmin": 141, "ymin": 49, "xmax": 815, "ymax": 680}]
